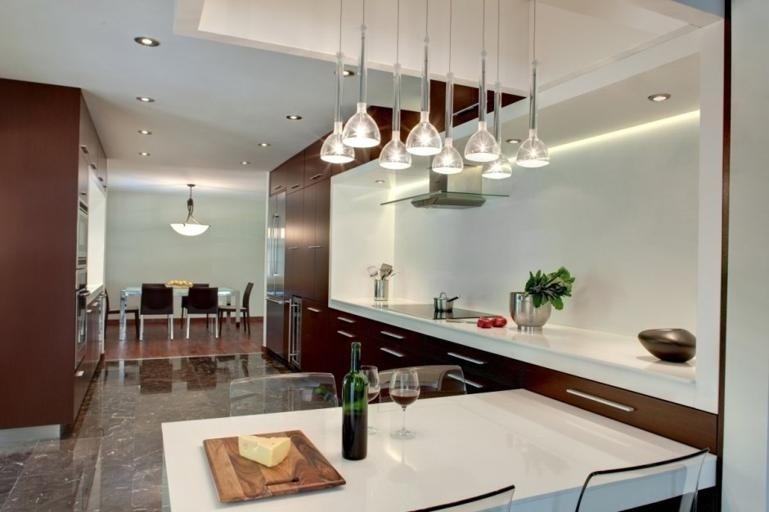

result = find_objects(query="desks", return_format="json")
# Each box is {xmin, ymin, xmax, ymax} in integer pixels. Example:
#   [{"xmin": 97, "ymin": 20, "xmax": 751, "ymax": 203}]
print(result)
[{"xmin": 118, "ymin": 287, "xmax": 240, "ymax": 340}]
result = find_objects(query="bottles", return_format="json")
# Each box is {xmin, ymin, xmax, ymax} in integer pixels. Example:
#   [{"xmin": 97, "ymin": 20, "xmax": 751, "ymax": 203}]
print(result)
[{"xmin": 340, "ymin": 342, "xmax": 366, "ymax": 460}]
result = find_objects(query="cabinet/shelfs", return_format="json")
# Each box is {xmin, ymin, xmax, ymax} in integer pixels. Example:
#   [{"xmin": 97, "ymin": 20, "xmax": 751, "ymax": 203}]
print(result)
[
  {"xmin": 423, "ymin": 333, "xmax": 521, "ymax": 394},
  {"xmin": 286, "ymin": 131, "xmax": 331, "ymax": 193},
  {"xmin": 301, "ymin": 298, "xmax": 328, "ymax": 373},
  {"xmin": 269, "ymin": 162, "xmax": 286, "ymax": 195},
  {"xmin": 366, "ymin": 317, "xmax": 423, "ymax": 397},
  {"xmin": 328, "ymin": 307, "xmax": 366, "ymax": 398},
  {"xmin": 521, "ymin": 362, "xmax": 721, "ymax": 512},
  {"xmin": 284, "ymin": 178, "xmax": 330, "ymax": 303},
  {"xmin": 0, "ymin": 78, "xmax": 107, "ymax": 205}
]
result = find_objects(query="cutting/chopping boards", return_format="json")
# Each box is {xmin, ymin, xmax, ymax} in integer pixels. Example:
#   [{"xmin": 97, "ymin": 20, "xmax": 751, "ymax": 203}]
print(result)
[{"xmin": 204, "ymin": 430, "xmax": 346, "ymax": 501}]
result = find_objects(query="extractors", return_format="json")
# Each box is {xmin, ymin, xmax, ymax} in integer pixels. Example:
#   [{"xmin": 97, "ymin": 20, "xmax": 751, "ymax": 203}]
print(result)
[{"xmin": 381, "ymin": 167, "xmax": 508, "ymax": 208}]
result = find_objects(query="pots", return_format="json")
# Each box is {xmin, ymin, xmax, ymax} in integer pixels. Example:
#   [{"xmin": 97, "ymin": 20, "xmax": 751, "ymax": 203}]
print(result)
[
  {"xmin": 433, "ymin": 292, "xmax": 453, "ymax": 312},
  {"xmin": 510, "ymin": 292, "xmax": 551, "ymax": 330}
]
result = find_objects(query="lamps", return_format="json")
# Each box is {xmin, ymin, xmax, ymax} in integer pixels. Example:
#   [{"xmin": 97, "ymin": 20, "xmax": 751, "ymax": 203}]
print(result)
[{"xmin": 170, "ymin": 183, "xmax": 210, "ymax": 237}]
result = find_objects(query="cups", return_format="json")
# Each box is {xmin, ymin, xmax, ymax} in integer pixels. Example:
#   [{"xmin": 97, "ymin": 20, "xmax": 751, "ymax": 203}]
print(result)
[{"xmin": 374, "ymin": 280, "xmax": 388, "ymax": 300}]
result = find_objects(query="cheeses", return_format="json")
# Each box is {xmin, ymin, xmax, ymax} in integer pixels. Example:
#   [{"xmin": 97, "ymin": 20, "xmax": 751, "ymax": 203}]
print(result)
[{"xmin": 238, "ymin": 434, "xmax": 291, "ymax": 467}]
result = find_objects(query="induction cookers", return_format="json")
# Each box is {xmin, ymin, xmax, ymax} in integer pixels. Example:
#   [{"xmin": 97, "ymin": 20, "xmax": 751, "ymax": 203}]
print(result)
[{"xmin": 372, "ymin": 304, "xmax": 494, "ymax": 321}]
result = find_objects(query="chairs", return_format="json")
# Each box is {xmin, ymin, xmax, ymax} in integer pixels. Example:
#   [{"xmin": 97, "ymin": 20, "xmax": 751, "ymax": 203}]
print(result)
[
  {"xmin": 412, "ymin": 485, "xmax": 515, "ymax": 512},
  {"xmin": 104, "ymin": 289, "xmax": 139, "ymax": 340},
  {"xmin": 378, "ymin": 364, "xmax": 467, "ymax": 403},
  {"xmin": 139, "ymin": 288, "xmax": 174, "ymax": 340},
  {"xmin": 142, "ymin": 283, "xmax": 165, "ymax": 287},
  {"xmin": 576, "ymin": 448, "xmax": 710, "ymax": 512},
  {"xmin": 186, "ymin": 287, "xmax": 218, "ymax": 339},
  {"xmin": 229, "ymin": 372, "xmax": 338, "ymax": 416},
  {"xmin": 218, "ymin": 283, "xmax": 254, "ymax": 337},
  {"xmin": 181, "ymin": 284, "xmax": 209, "ymax": 328}
]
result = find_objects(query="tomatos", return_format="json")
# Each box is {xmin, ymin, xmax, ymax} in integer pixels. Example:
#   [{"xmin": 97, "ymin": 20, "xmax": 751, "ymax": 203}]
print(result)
[{"xmin": 477, "ymin": 317, "xmax": 506, "ymax": 328}]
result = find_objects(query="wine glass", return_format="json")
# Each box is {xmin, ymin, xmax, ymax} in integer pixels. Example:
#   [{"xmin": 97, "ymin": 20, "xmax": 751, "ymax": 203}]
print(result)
[
  {"xmin": 389, "ymin": 369, "xmax": 421, "ymax": 441},
  {"xmin": 359, "ymin": 364, "xmax": 380, "ymax": 403}
]
